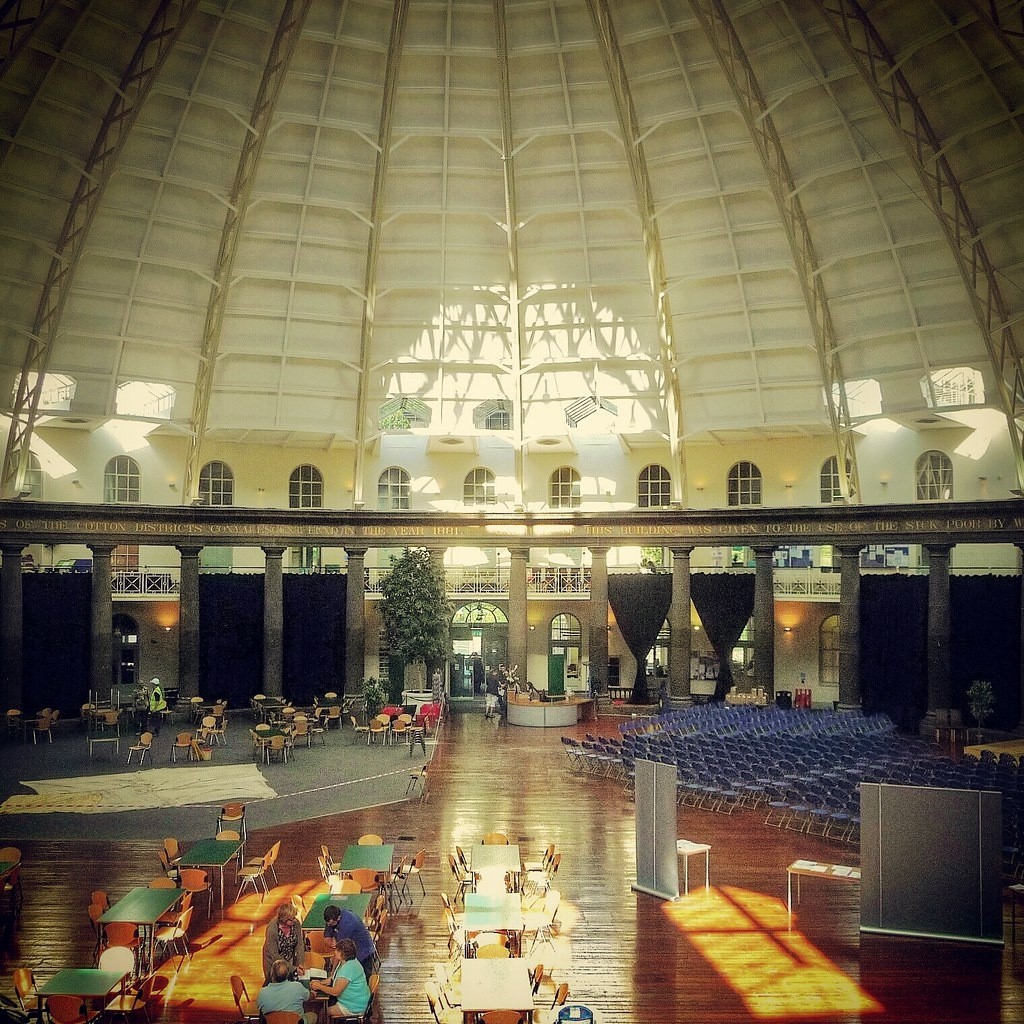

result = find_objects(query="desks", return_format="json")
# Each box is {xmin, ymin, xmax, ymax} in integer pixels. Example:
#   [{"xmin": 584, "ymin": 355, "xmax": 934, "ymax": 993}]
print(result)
[
  {"xmin": 95, "ymin": 886, "xmax": 187, "ymax": 975},
  {"xmin": 88, "ymin": 689, "xmax": 121, "ymax": 714},
  {"xmin": 34, "ymin": 968, "xmax": 127, "ymax": 1024},
  {"xmin": 785, "ymin": 860, "xmax": 861, "ymax": 933},
  {"xmin": 469, "ymin": 845, "xmax": 522, "ymax": 893},
  {"xmin": 461, "ymin": 957, "xmax": 535, "ymax": 1024},
  {"xmin": 463, "ymin": 893, "xmax": 523, "ymax": 958},
  {"xmin": 676, "ymin": 839, "xmax": 711, "ymax": 895},
  {"xmin": 11, "ymin": 713, "xmax": 45, "ymax": 740},
  {"xmin": 255, "ymin": 956, "xmax": 333, "ymax": 1024},
  {"xmin": 175, "ymin": 840, "xmax": 247, "ymax": 910},
  {"xmin": 253, "ymin": 729, "xmax": 285, "ymax": 761},
  {"xmin": 87, "ymin": 708, "xmax": 120, "ymax": 732},
  {"xmin": 83, "ymin": 730, "xmax": 118, "ymax": 756},
  {"xmin": 300, "ymin": 893, "xmax": 372, "ymax": 942},
  {"xmin": 338, "ymin": 844, "xmax": 395, "ymax": 917},
  {"xmin": 251, "ymin": 698, "xmax": 286, "ymax": 721},
  {"xmin": 316, "ymin": 698, "xmax": 342, "ymax": 726},
  {"xmin": 195, "ymin": 700, "xmax": 222, "ymax": 718}
]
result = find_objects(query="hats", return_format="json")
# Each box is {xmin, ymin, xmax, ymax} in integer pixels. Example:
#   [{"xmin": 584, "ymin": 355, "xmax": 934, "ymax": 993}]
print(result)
[
  {"xmin": 138, "ymin": 680, "xmax": 145, "ymax": 686},
  {"xmin": 150, "ymin": 678, "xmax": 160, "ymax": 684}
]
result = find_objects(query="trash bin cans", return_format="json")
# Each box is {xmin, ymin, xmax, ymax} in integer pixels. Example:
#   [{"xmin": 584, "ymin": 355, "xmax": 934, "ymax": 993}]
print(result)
[
  {"xmin": 775, "ymin": 690, "xmax": 791, "ymax": 709},
  {"xmin": 794, "ymin": 687, "xmax": 812, "ymax": 710},
  {"xmin": 162, "ymin": 686, "xmax": 180, "ymax": 710}
]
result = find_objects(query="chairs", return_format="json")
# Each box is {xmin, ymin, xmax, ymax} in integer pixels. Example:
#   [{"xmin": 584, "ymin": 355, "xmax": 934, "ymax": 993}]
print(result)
[
  {"xmin": 560, "ymin": 700, "xmax": 1024, "ymax": 900},
  {"xmin": 0, "ymin": 690, "xmax": 593, "ymax": 1024}
]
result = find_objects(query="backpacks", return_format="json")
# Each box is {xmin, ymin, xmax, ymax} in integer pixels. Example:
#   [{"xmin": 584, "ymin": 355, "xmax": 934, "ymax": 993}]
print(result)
[{"xmin": 136, "ymin": 688, "xmax": 149, "ymax": 710}]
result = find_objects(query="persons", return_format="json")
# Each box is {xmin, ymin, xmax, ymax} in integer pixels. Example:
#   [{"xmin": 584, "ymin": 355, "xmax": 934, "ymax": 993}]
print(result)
[
  {"xmin": 486, "ymin": 672, "xmax": 505, "ymax": 718},
  {"xmin": 132, "ymin": 680, "xmax": 151, "ymax": 736},
  {"xmin": 262, "ymin": 903, "xmax": 305, "ymax": 983},
  {"xmin": 525, "ymin": 682, "xmax": 540, "ymax": 701},
  {"xmin": 257, "ymin": 959, "xmax": 316, "ymax": 1024},
  {"xmin": 149, "ymin": 677, "xmax": 167, "ymax": 736},
  {"xmin": 324, "ymin": 905, "xmax": 373, "ymax": 984},
  {"xmin": 310, "ymin": 938, "xmax": 371, "ymax": 1024}
]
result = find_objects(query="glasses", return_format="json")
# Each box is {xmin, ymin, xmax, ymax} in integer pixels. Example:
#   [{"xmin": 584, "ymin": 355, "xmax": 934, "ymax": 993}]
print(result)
[{"xmin": 287, "ymin": 917, "xmax": 295, "ymax": 922}]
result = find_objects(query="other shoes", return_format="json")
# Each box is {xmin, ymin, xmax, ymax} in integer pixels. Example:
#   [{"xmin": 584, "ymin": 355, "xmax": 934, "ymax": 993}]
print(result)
[
  {"xmin": 135, "ymin": 732, "xmax": 142, "ymax": 735},
  {"xmin": 151, "ymin": 732, "xmax": 159, "ymax": 737}
]
